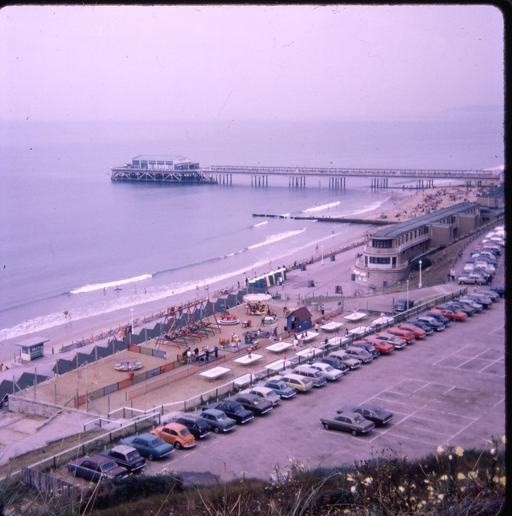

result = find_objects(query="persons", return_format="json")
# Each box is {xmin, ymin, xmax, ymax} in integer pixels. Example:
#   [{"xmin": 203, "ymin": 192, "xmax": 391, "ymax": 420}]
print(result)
[{"xmin": 163, "ymin": 301, "xmax": 351, "ymax": 365}]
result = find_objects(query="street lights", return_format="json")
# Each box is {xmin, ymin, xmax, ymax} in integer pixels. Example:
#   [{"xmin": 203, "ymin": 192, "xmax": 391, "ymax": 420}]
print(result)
[{"xmin": 418, "ymin": 260, "xmax": 423, "ymax": 289}]
